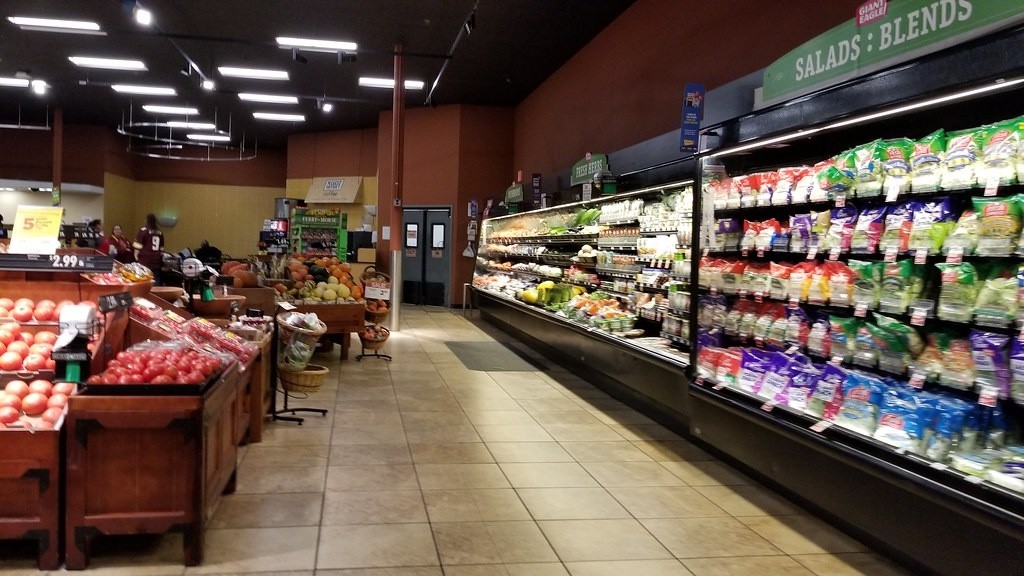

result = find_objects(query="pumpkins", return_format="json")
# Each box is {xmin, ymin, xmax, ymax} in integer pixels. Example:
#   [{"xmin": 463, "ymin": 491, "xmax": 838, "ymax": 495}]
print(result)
[
  {"xmin": 221, "ymin": 260, "xmax": 258, "ymax": 287},
  {"xmin": 274, "ymin": 263, "xmax": 315, "ymax": 299}
]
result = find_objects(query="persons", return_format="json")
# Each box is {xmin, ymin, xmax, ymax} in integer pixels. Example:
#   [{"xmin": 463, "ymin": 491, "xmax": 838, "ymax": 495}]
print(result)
[
  {"xmin": 88, "ymin": 219, "xmax": 110, "ymax": 255},
  {"xmin": 109, "ymin": 224, "xmax": 133, "ymax": 264},
  {"xmin": 133, "ymin": 213, "xmax": 164, "ymax": 286},
  {"xmin": 195, "ymin": 239, "xmax": 222, "ymax": 263}
]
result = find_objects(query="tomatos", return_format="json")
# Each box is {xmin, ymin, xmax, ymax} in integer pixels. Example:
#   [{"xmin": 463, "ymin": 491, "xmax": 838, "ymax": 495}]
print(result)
[
  {"xmin": 87, "ymin": 348, "xmax": 219, "ymax": 384},
  {"xmin": 0, "ymin": 297, "xmax": 100, "ymax": 424}
]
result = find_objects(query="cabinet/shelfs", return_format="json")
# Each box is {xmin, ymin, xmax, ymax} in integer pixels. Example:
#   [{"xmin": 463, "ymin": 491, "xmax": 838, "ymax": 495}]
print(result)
[{"xmin": 0, "ymin": 208, "xmax": 392, "ymax": 576}]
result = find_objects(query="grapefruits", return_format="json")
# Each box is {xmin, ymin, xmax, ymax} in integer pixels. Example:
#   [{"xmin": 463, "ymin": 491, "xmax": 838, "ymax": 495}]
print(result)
[{"xmin": 314, "ymin": 257, "xmax": 361, "ymax": 299}]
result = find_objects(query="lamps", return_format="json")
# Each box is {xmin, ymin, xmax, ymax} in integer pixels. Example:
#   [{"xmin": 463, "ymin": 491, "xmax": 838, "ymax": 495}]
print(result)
[{"xmin": 1, "ymin": 4, "xmax": 425, "ymax": 145}]
